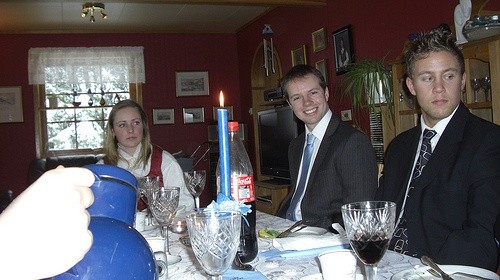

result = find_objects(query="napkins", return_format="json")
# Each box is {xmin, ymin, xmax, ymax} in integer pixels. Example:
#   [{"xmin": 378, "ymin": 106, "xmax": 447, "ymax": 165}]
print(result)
[{"xmin": 273, "ymin": 232, "xmax": 350, "ymax": 251}]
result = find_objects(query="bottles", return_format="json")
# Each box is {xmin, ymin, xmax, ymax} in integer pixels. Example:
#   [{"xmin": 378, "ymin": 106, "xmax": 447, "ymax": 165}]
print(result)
[{"xmin": 216, "ymin": 121, "xmax": 258, "ymax": 262}]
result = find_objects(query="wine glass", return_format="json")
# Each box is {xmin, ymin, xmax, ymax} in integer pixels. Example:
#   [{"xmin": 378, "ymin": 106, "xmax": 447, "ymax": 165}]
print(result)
[
  {"xmin": 185, "ymin": 211, "xmax": 241, "ymax": 280},
  {"xmin": 183, "ymin": 171, "xmax": 206, "ymax": 211},
  {"xmin": 341, "ymin": 201, "xmax": 396, "ymax": 280},
  {"xmin": 136, "ymin": 176, "xmax": 160, "ymax": 230},
  {"xmin": 146, "ymin": 186, "xmax": 183, "ymax": 266}
]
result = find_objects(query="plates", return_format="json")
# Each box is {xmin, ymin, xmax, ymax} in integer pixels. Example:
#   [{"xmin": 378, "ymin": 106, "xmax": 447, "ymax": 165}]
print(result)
[
  {"xmin": 259, "ymin": 227, "xmax": 328, "ymax": 239},
  {"xmin": 142, "ymin": 205, "xmax": 186, "ymax": 217},
  {"xmin": 299, "ymin": 272, "xmax": 367, "ymax": 280},
  {"xmin": 390, "ymin": 264, "xmax": 499, "ymax": 280}
]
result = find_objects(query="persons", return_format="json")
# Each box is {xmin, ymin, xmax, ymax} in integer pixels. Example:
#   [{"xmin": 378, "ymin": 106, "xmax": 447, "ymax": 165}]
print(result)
[
  {"xmin": 274, "ymin": 63, "xmax": 379, "ymax": 233},
  {"xmin": 95, "ymin": 99, "xmax": 194, "ymax": 230},
  {"xmin": 0, "ymin": 165, "xmax": 94, "ymax": 279},
  {"xmin": 372, "ymin": 29, "xmax": 500, "ymax": 272}
]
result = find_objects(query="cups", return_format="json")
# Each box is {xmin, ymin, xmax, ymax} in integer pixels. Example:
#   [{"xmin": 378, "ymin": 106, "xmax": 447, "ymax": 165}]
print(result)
[
  {"xmin": 145, "ymin": 237, "xmax": 166, "ymax": 262},
  {"xmin": 481, "ymin": 76, "xmax": 491, "ymax": 102},
  {"xmin": 471, "ymin": 78, "xmax": 481, "ymax": 102},
  {"xmin": 317, "ymin": 248, "xmax": 358, "ymax": 280},
  {"xmin": 134, "ymin": 211, "xmax": 147, "ymax": 232}
]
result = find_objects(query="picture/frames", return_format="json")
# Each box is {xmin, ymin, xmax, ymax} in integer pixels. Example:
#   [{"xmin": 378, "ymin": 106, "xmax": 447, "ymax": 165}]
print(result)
[
  {"xmin": 207, "ymin": 124, "xmax": 247, "ymax": 142},
  {"xmin": 332, "ymin": 24, "xmax": 355, "ymax": 76},
  {"xmin": 312, "ymin": 28, "xmax": 328, "ymax": 53},
  {"xmin": 0, "ymin": 85, "xmax": 25, "ymax": 125},
  {"xmin": 182, "ymin": 106, "xmax": 206, "ymax": 124},
  {"xmin": 291, "ymin": 43, "xmax": 307, "ymax": 67},
  {"xmin": 212, "ymin": 105, "xmax": 234, "ymax": 122},
  {"xmin": 315, "ymin": 58, "xmax": 330, "ymax": 90},
  {"xmin": 174, "ymin": 69, "xmax": 211, "ymax": 98}
]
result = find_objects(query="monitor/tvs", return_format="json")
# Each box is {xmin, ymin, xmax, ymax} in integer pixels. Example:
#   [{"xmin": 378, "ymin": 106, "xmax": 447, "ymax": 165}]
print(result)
[{"xmin": 256, "ymin": 106, "xmax": 303, "ymax": 180}]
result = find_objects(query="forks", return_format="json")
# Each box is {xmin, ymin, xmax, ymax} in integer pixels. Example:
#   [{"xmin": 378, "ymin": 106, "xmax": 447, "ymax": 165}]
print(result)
[{"xmin": 427, "ymin": 269, "xmax": 493, "ymax": 280}]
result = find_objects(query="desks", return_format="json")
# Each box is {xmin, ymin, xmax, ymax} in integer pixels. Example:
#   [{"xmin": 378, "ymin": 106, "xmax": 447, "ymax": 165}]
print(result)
[{"xmin": 136, "ymin": 208, "xmax": 428, "ymax": 280}]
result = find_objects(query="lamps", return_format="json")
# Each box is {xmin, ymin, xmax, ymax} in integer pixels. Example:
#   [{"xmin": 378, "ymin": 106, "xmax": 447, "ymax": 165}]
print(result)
[{"xmin": 80, "ymin": 2, "xmax": 108, "ymax": 23}]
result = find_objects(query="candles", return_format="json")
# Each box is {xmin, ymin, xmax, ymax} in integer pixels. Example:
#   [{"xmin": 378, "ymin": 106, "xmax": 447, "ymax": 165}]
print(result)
[{"xmin": 217, "ymin": 105, "xmax": 231, "ymax": 200}]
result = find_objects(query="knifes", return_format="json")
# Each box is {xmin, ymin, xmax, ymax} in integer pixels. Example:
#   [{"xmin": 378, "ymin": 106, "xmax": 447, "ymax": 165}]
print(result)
[
  {"xmin": 420, "ymin": 255, "xmax": 453, "ymax": 280},
  {"xmin": 278, "ymin": 219, "xmax": 305, "ymax": 239}
]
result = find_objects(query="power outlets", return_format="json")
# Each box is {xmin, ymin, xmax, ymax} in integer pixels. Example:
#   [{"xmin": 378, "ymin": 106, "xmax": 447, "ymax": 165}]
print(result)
[{"xmin": 341, "ymin": 110, "xmax": 352, "ymax": 121}]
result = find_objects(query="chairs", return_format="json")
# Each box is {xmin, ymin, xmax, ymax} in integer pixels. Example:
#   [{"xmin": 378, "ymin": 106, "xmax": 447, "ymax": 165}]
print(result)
[{"xmin": 24, "ymin": 154, "xmax": 106, "ymax": 188}]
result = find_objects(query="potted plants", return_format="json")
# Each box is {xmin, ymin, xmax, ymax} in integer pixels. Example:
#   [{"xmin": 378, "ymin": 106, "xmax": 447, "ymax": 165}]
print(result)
[{"xmin": 333, "ymin": 52, "xmax": 396, "ymax": 134}]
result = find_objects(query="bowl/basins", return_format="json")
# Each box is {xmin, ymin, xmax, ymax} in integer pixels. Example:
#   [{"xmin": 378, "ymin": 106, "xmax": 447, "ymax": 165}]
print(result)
[{"xmin": 462, "ymin": 14, "xmax": 500, "ymax": 41}]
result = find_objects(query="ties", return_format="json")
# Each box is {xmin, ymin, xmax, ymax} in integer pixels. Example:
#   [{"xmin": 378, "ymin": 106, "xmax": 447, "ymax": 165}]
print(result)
[
  {"xmin": 286, "ymin": 133, "xmax": 316, "ymax": 221},
  {"xmin": 391, "ymin": 129, "xmax": 437, "ymax": 255}
]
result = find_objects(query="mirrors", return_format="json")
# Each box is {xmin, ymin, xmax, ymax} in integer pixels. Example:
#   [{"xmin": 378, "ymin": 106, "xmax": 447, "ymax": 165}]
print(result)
[{"xmin": 151, "ymin": 107, "xmax": 176, "ymax": 125}]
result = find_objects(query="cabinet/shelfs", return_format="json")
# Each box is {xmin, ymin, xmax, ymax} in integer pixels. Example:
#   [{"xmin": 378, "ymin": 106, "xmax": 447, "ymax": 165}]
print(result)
[
  {"xmin": 393, "ymin": 34, "xmax": 500, "ymax": 136},
  {"xmin": 255, "ymin": 179, "xmax": 290, "ymax": 215}
]
